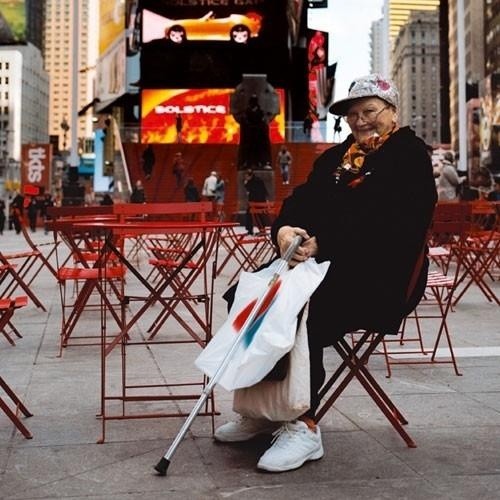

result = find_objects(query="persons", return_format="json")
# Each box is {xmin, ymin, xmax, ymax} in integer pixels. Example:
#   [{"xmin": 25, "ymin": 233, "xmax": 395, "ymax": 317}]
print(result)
[
  {"xmin": 215, "ymin": 74, "xmax": 438, "ymax": 473},
  {"xmin": 142, "ymin": 144, "xmax": 155, "ymax": 181},
  {"xmin": 129, "ymin": 180, "xmax": 145, "ymax": 203},
  {"xmin": 176, "ymin": 113, "xmax": 182, "ymax": 144},
  {"xmin": 184, "ymin": 171, "xmax": 225, "ymax": 223},
  {"xmin": 437, "ymin": 152, "xmax": 500, "ymax": 230},
  {"xmin": 0, "ymin": 194, "xmax": 53, "ymax": 235},
  {"xmin": 275, "ymin": 145, "xmax": 293, "ymax": 184},
  {"xmin": 242, "ymin": 168, "xmax": 266, "ymax": 235},
  {"xmin": 172, "ymin": 152, "xmax": 184, "ymax": 188}
]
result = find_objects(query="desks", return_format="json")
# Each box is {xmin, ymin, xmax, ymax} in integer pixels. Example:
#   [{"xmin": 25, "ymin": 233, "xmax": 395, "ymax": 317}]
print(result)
[{"xmin": 72, "ymin": 220, "xmax": 240, "ymax": 444}]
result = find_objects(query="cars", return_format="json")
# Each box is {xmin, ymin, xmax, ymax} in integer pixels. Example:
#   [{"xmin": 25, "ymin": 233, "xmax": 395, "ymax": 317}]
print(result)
[{"xmin": 165, "ymin": 11, "xmax": 263, "ymax": 49}]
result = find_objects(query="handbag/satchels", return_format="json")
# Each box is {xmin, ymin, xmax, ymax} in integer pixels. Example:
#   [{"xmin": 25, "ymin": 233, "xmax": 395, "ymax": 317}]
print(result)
[{"xmin": 223, "ymin": 258, "xmax": 312, "ymax": 422}]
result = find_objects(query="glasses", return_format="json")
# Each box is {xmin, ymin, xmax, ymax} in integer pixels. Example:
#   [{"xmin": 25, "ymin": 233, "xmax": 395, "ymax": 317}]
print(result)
[{"xmin": 343, "ymin": 104, "xmax": 390, "ymax": 123}]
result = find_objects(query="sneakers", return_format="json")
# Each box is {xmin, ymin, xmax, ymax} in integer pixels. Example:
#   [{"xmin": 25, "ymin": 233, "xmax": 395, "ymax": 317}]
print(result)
[
  {"xmin": 257, "ymin": 419, "xmax": 324, "ymax": 472},
  {"xmin": 214, "ymin": 415, "xmax": 275, "ymax": 442}
]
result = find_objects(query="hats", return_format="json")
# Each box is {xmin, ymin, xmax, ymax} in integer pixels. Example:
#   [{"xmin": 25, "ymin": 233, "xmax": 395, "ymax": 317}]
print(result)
[{"xmin": 329, "ymin": 73, "xmax": 401, "ymax": 116}]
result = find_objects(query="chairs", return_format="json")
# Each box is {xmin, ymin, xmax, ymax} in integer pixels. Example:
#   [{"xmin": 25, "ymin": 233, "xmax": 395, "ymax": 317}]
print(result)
[
  {"xmin": 313, "ymin": 200, "xmax": 439, "ymax": 447},
  {"xmin": 0, "ymin": 376, "xmax": 34, "ymax": 439},
  {"xmin": 119, "ymin": 219, "xmax": 221, "ymax": 420}
]
